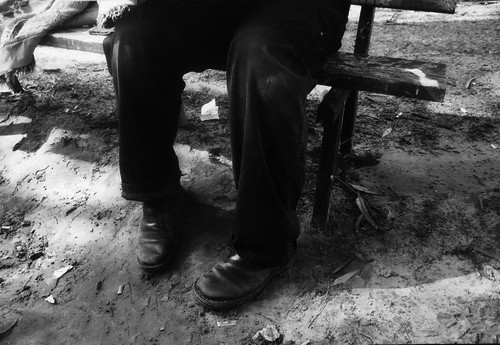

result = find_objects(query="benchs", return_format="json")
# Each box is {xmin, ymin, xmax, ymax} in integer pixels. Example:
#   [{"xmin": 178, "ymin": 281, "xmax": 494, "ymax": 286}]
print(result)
[{"xmin": 39, "ymin": 0, "xmax": 456, "ymax": 228}]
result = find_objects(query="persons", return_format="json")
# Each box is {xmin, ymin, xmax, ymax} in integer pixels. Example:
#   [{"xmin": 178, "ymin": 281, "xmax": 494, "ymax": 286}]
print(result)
[{"xmin": 101, "ymin": 0, "xmax": 352, "ymax": 310}]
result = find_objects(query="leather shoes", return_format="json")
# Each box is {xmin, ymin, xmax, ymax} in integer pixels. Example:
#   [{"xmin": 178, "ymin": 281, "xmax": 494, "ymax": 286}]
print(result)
[
  {"xmin": 192, "ymin": 239, "xmax": 298, "ymax": 309},
  {"xmin": 137, "ymin": 185, "xmax": 186, "ymax": 271}
]
